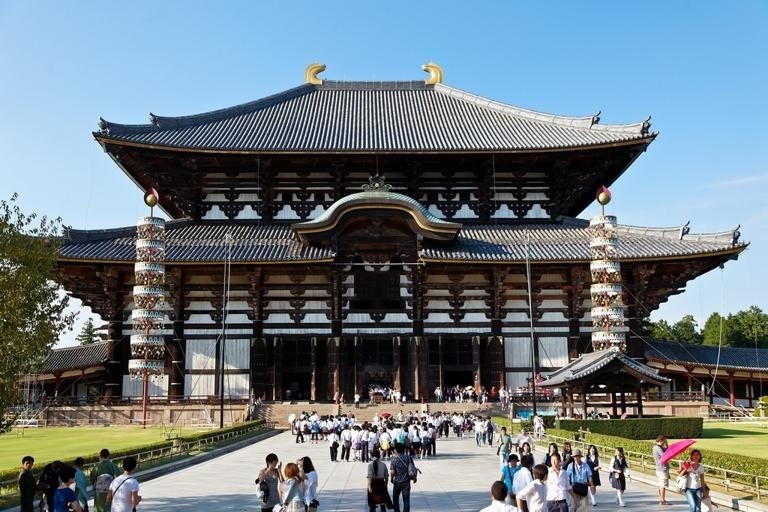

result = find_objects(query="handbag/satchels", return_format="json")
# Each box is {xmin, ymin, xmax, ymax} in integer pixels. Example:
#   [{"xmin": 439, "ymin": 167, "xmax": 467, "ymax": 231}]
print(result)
[
  {"xmin": 572, "ymin": 482, "xmax": 588, "ymax": 497},
  {"xmin": 272, "ymin": 498, "xmax": 319, "ymax": 512},
  {"xmin": 496, "ymin": 447, "xmax": 501, "ymax": 455},
  {"xmin": 332, "ymin": 440, "xmax": 392, "ymax": 451},
  {"xmin": 408, "ymin": 463, "xmax": 422, "ymax": 483},
  {"xmin": 255, "ymin": 478, "xmax": 269, "ymax": 498}
]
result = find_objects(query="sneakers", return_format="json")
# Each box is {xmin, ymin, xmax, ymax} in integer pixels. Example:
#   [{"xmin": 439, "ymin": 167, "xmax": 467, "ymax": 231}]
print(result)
[
  {"xmin": 332, "ymin": 456, "xmax": 429, "ymax": 462},
  {"xmin": 615, "ymin": 497, "xmax": 626, "ymax": 508},
  {"xmin": 591, "ymin": 500, "xmax": 597, "ymax": 506}
]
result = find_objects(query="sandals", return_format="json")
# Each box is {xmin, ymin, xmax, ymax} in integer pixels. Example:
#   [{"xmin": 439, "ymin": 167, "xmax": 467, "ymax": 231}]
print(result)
[{"xmin": 659, "ymin": 500, "xmax": 671, "ymax": 506}]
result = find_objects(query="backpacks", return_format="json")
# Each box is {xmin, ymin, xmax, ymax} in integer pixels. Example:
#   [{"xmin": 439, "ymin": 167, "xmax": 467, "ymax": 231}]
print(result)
[{"xmin": 397, "ymin": 433, "xmax": 404, "ymax": 443}]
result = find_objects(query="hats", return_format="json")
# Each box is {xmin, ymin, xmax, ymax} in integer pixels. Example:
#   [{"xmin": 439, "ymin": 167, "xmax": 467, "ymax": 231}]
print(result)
[
  {"xmin": 571, "ymin": 450, "xmax": 583, "ymax": 458},
  {"xmin": 507, "ymin": 454, "xmax": 520, "ymax": 462}
]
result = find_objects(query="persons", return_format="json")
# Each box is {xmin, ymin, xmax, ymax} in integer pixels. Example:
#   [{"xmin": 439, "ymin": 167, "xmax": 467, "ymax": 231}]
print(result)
[
  {"xmin": 678, "ymin": 449, "xmax": 718, "ymax": 511},
  {"xmin": 89, "ymin": 448, "xmax": 122, "ymax": 511},
  {"xmin": 533, "ymin": 412, "xmax": 546, "ymax": 439},
  {"xmin": 259, "ymin": 454, "xmax": 318, "ymax": 512},
  {"xmin": 369, "ymin": 386, "xmax": 414, "ymax": 407},
  {"xmin": 17, "ymin": 454, "xmax": 37, "ymax": 512},
  {"xmin": 515, "ymin": 386, "xmax": 562, "ymax": 403},
  {"xmin": 353, "ymin": 391, "xmax": 360, "ymax": 409},
  {"xmin": 72, "ymin": 456, "xmax": 90, "ymax": 511},
  {"xmin": 333, "ymin": 389, "xmax": 344, "ymax": 406},
  {"xmin": 328, "ymin": 410, "xmax": 498, "ymax": 463},
  {"xmin": 38, "ymin": 459, "xmax": 66, "ymax": 512},
  {"xmin": 481, "ymin": 427, "xmax": 632, "ymax": 511},
  {"xmin": 554, "ymin": 410, "xmax": 611, "ymax": 427},
  {"xmin": 653, "ymin": 435, "xmax": 674, "ymax": 505},
  {"xmin": 434, "ymin": 385, "xmax": 509, "ymax": 411},
  {"xmin": 290, "ymin": 410, "xmax": 356, "ymax": 444},
  {"xmin": 366, "ymin": 443, "xmax": 414, "ymax": 512},
  {"xmin": 106, "ymin": 456, "xmax": 142, "ymax": 511},
  {"xmin": 256, "ymin": 395, "xmax": 265, "ymax": 407},
  {"xmin": 51, "ymin": 465, "xmax": 83, "ymax": 511}
]
